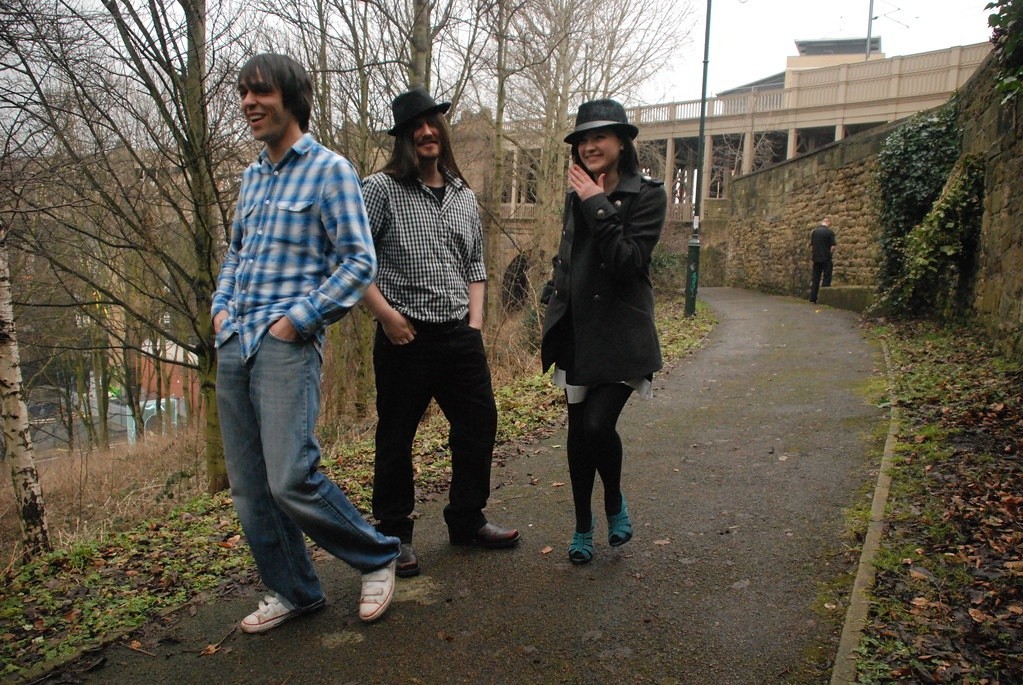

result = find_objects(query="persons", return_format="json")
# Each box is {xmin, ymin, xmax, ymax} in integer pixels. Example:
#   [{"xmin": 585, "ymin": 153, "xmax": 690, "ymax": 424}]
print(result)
[
  {"xmin": 207, "ymin": 48, "xmax": 403, "ymax": 636},
  {"xmin": 810, "ymin": 218, "xmax": 838, "ymax": 304},
  {"xmin": 537, "ymin": 98, "xmax": 670, "ymax": 569},
  {"xmin": 353, "ymin": 89, "xmax": 523, "ymax": 578}
]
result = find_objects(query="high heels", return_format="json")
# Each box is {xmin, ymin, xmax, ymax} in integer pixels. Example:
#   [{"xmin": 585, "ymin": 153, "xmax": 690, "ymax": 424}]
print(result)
[
  {"xmin": 569, "ymin": 514, "xmax": 596, "ymax": 563},
  {"xmin": 606, "ymin": 491, "xmax": 633, "ymax": 547}
]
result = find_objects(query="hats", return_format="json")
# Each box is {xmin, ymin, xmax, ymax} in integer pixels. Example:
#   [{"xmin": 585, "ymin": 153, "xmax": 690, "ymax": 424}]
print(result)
[
  {"xmin": 387, "ymin": 89, "xmax": 451, "ymax": 136},
  {"xmin": 564, "ymin": 99, "xmax": 639, "ymax": 144}
]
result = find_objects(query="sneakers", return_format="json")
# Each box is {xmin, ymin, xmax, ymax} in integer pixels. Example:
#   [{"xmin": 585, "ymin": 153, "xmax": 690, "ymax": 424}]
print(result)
[
  {"xmin": 359, "ymin": 560, "xmax": 396, "ymax": 622},
  {"xmin": 242, "ymin": 591, "xmax": 326, "ymax": 633}
]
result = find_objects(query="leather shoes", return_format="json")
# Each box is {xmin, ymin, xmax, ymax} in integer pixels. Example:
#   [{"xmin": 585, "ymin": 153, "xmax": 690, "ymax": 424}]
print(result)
[
  {"xmin": 449, "ymin": 522, "xmax": 520, "ymax": 547},
  {"xmin": 394, "ymin": 543, "xmax": 421, "ymax": 577}
]
